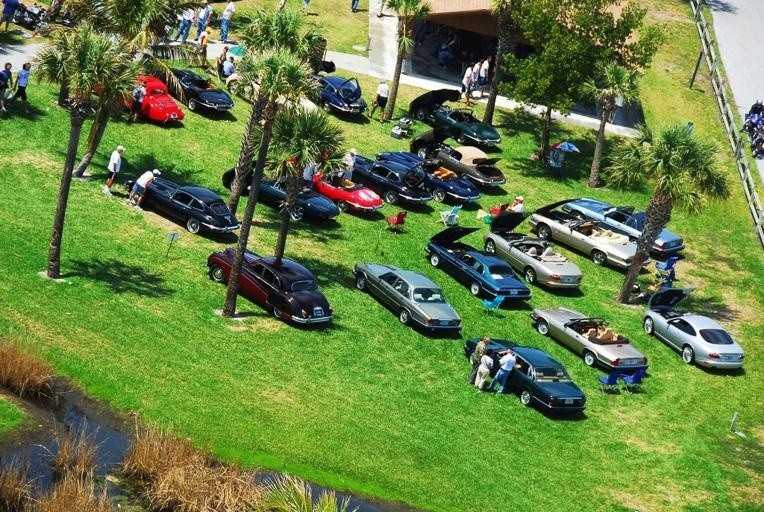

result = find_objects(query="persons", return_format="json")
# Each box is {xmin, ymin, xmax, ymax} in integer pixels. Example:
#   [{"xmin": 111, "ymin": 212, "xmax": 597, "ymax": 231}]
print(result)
[
  {"xmin": 608, "ymin": 94, "xmax": 624, "ymax": 124},
  {"xmin": 597, "ymin": 322, "xmax": 605, "ymax": 336},
  {"xmin": 507, "ymin": 196, "xmax": 523, "ymax": 212},
  {"xmin": 302, "ymin": 0, "xmax": 310, "ymax": 16},
  {"xmin": 487, "ymin": 349, "xmax": 517, "ymax": 393},
  {"xmin": 278, "ymin": 0, "xmax": 286, "ymax": 11},
  {"xmin": 467, "ymin": 335, "xmax": 489, "ymax": 383},
  {"xmin": 101, "ymin": 144, "xmax": 125, "ymax": 195},
  {"xmin": 11, "ymin": 63, "xmax": 30, "ymax": 114},
  {"xmin": 128, "ymin": 79, "xmax": 146, "ymax": 123},
  {"xmin": 351, "ymin": 0, "xmax": 358, "ymax": 13},
  {"xmin": 413, "ymin": 20, "xmax": 494, "ymax": 107},
  {"xmin": 127, "ymin": 168, "xmax": 161, "ymax": 211},
  {"xmin": 376, "ymin": 0, "xmax": 387, "ymax": 18},
  {"xmin": 548, "ymin": 148, "xmax": 566, "ymax": 180},
  {"xmin": 302, "ymin": 144, "xmax": 357, "ymax": 191},
  {"xmin": 0, "ymin": 0, "xmax": 71, "ymax": 39},
  {"xmin": 739, "ymin": 98, "xmax": 764, "ymax": 133},
  {"xmin": 161, "ymin": 1, "xmax": 235, "ymax": 78},
  {"xmin": 367, "ymin": 78, "xmax": 389, "ymax": 123},
  {"xmin": 601, "ymin": 98, "xmax": 608, "ymax": 121},
  {"xmin": 473, "ymin": 349, "xmax": 494, "ymax": 390},
  {"xmin": 0, "ymin": 62, "xmax": 13, "ymax": 113}
]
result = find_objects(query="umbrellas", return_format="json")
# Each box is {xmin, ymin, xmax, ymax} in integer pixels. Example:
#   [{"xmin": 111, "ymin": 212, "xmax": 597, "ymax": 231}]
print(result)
[
  {"xmin": 550, "ymin": 141, "xmax": 581, "ymax": 153},
  {"xmin": 228, "ymin": 46, "xmax": 247, "ymax": 57}
]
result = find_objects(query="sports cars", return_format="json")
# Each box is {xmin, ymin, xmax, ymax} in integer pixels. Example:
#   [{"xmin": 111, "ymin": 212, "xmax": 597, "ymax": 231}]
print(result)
[
  {"xmin": 151, "ymin": 68, "xmax": 234, "ymax": 113},
  {"xmin": 529, "ymin": 306, "xmax": 649, "ymax": 373},
  {"xmin": 222, "ymin": 158, "xmax": 339, "ymax": 222},
  {"xmin": 374, "ymin": 151, "xmax": 481, "ymax": 204},
  {"xmin": 285, "ymin": 141, "xmax": 385, "ymax": 214},
  {"xmin": 409, "ymin": 90, "xmax": 501, "ymax": 147},
  {"xmin": 528, "ymin": 198, "xmax": 651, "ymax": 270},
  {"xmin": 562, "ymin": 196, "xmax": 685, "ymax": 257},
  {"xmin": 483, "ymin": 211, "xmax": 582, "ymax": 290}
]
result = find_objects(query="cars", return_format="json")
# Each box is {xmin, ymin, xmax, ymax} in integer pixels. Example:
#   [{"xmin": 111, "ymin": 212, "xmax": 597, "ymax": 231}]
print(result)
[
  {"xmin": 463, "ymin": 337, "xmax": 586, "ymax": 412},
  {"xmin": 642, "ymin": 287, "xmax": 745, "ymax": 370},
  {"xmin": 409, "ymin": 124, "xmax": 506, "ymax": 187},
  {"xmin": 423, "ymin": 226, "xmax": 531, "ymax": 301},
  {"xmin": 350, "ymin": 155, "xmax": 433, "ymax": 206},
  {"xmin": 90, "ymin": 63, "xmax": 184, "ymax": 125},
  {"xmin": 225, "ymin": 69, "xmax": 321, "ymax": 121},
  {"xmin": 352, "ymin": 263, "xmax": 462, "ymax": 333},
  {"xmin": 300, "ymin": 61, "xmax": 367, "ymax": 117},
  {"xmin": 206, "ymin": 246, "xmax": 333, "ymax": 326},
  {"xmin": 123, "ymin": 177, "xmax": 239, "ymax": 235}
]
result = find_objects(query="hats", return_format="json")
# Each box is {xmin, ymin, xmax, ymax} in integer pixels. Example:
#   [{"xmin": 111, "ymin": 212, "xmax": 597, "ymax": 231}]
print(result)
[
  {"xmin": 117, "ymin": 145, "xmax": 125, "ymax": 151},
  {"xmin": 350, "ymin": 149, "xmax": 357, "ymax": 155},
  {"xmin": 153, "ymin": 169, "xmax": 161, "ymax": 175},
  {"xmin": 516, "ymin": 195, "xmax": 523, "ymax": 201}
]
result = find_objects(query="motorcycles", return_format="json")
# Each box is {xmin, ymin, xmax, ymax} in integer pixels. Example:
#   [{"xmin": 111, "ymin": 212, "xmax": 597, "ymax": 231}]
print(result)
[
  {"xmin": 11, "ymin": 4, "xmax": 50, "ymax": 37},
  {"xmin": 750, "ymin": 129, "xmax": 764, "ymax": 158},
  {"xmin": 745, "ymin": 111, "xmax": 764, "ymax": 140}
]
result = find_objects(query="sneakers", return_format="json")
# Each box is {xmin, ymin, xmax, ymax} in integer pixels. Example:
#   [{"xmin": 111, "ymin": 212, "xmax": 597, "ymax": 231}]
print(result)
[
  {"xmin": 103, "ymin": 188, "xmax": 113, "ymax": 195},
  {"xmin": 135, "ymin": 205, "xmax": 143, "ymax": 211},
  {"xmin": 127, "ymin": 198, "xmax": 133, "ymax": 205}
]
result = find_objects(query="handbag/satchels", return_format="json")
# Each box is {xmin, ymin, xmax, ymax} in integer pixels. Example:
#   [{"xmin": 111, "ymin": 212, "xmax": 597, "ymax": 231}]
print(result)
[{"xmin": 469, "ymin": 354, "xmax": 479, "ymax": 369}]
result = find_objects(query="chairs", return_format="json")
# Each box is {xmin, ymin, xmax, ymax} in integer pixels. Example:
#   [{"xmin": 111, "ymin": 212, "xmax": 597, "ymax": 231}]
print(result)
[
  {"xmin": 599, "ymin": 370, "xmax": 623, "ymax": 394},
  {"xmin": 413, "ymin": 293, "xmax": 440, "ymax": 301},
  {"xmin": 432, "ymin": 167, "xmax": 455, "ymax": 180},
  {"xmin": 482, "ymin": 295, "xmax": 506, "ymax": 315},
  {"xmin": 436, "ymin": 204, "xmax": 463, "ymax": 227},
  {"xmin": 622, "ymin": 368, "xmax": 645, "ymax": 394},
  {"xmin": 385, "ymin": 210, "xmax": 407, "ymax": 233},
  {"xmin": 536, "ymin": 371, "xmax": 567, "ymax": 379},
  {"xmin": 655, "ymin": 256, "xmax": 679, "ymax": 272},
  {"xmin": 581, "ymin": 327, "xmax": 615, "ymax": 342},
  {"xmin": 525, "ymin": 246, "xmax": 556, "ymax": 257},
  {"xmin": 655, "ymin": 271, "xmax": 675, "ymax": 290},
  {"xmin": 591, "ymin": 229, "xmax": 613, "ymax": 237}
]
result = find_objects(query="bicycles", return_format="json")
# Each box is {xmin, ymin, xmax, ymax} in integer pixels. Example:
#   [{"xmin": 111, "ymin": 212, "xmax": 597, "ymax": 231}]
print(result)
[{"xmin": 70, "ymin": 93, "xmax": 95, "ymax": 117}]
